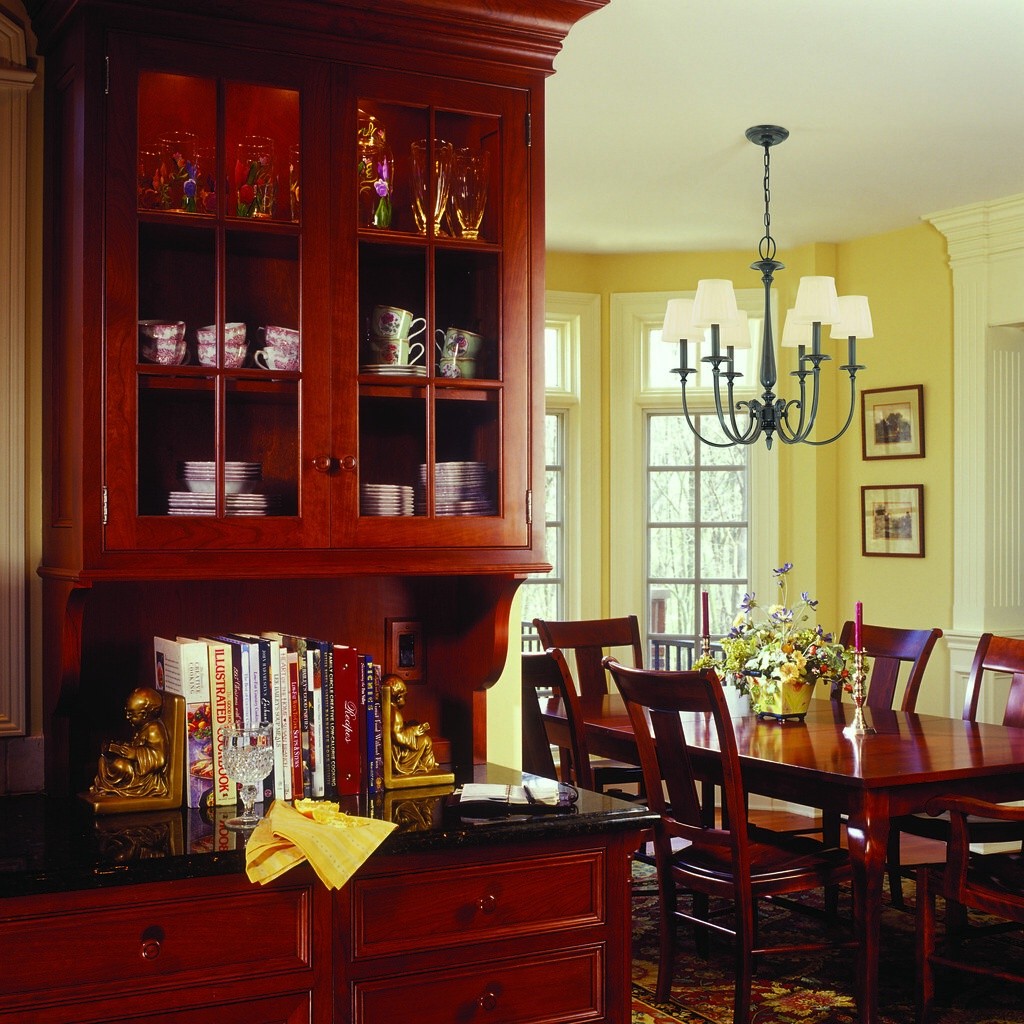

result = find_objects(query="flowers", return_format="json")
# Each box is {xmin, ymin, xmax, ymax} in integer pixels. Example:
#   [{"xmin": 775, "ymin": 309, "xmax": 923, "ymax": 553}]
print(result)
[{"xmin": 691, "ymin": 564, "xmax": 869, "ymax": 713}]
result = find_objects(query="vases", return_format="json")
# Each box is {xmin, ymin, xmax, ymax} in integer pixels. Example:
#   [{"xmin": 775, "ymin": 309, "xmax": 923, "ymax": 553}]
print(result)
[{"xmin": 741, "ymin": 667, "xmax": 819, "ymax": 722}]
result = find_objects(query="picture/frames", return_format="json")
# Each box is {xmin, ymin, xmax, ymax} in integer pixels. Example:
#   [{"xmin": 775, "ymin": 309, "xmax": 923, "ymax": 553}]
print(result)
[
  {"xmin": 861, "ymin": 384, "xmax": 927, "ymax": 461},
  {"xmin": 860, "ymin": 482, "xmax": 926, "ymax": 559}
]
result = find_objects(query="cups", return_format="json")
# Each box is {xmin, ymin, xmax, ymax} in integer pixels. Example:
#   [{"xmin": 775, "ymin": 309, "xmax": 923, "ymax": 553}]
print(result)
[
  {"xmin": 138, "ymin": 127, "xmax": 300, "ymax": 221},
  {"xmin": 366, "ymin": 304, "xmax": 426, "ymax": 365},
  {"xmin": 408, "ymin": 137, "xmax": 487, "ymax": 241},
  {"xmin": 435, "ymin": 327, "xmax": 485, "ymax": 377},
  {"xmin": 138, "ymin": 315, "xmax": 299, "ymax": 370}
]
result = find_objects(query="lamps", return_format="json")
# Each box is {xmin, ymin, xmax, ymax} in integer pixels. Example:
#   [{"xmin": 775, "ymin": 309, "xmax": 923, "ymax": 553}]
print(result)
[{"xmin": 661, "ymin": 126, "xmax": 877, "ymax": 452}]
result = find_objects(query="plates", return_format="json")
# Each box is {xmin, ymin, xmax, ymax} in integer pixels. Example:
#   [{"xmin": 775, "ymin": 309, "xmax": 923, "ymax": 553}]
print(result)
[
  {"xmin": 168, "ymin": 491, "xmax": 266, "ymax": 517},
  {"xmin": 360, "ymin": 365, "xmax": 426, "ymax": 376},
  {"xmin": 360, "ymin": 462, "xmax": 496, "ymax": 516}
]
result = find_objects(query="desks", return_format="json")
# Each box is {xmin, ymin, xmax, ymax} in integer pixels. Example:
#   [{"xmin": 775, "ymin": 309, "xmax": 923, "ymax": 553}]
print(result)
[{"xmin": 537, "ymin": 672, "xmax": 1024, "ymax": 1024}]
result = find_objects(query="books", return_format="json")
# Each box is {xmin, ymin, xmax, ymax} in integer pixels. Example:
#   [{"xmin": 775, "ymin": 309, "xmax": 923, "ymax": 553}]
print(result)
[
  {"xmin": 460, "ymin": 783, "xmax": 544, "ymax": 805},
  {"xmin": 154, "ymin": 631, "xmax": 383, "ymax": 808}
]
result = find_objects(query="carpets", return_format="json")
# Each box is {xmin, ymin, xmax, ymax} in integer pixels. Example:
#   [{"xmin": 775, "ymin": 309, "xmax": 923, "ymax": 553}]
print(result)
[{"xmin": 630, "ymin": 814, "xmax": 1024, "ymax": 1024}]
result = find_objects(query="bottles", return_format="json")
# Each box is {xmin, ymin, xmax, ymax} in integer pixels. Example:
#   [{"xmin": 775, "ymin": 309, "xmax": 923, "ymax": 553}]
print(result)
[{"xmin": 356, "ymin": 107, "xmax": 395, "ymax": 229}]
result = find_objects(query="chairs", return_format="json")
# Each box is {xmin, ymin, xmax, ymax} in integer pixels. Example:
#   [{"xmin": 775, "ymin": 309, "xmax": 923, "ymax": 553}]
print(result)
[
  {"xmin": 521, "ymin": 648, "xmax": 670, "ymax": 934},
  {"xmin": 600, "ymin": 654, "xmax": 852, "ymax": 1024},
  {"xmin": 882, "ymin": 633, "xmax": 1024, "ymax": 906},
  {"xmin": 531, "ymin": 615, "xmax": 646, "ymax": 799},
  {"xmin": 831, "ymin": 614, "xmax": 942, "ymax": 709},
  {"xmin": 897, "ymin": 794, "xmax": 1024, "ymax": 1024}
]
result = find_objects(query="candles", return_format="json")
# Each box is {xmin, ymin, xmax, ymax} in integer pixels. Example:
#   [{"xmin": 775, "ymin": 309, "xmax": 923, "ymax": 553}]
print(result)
[
  {"xmin": 701, "ymin": 591, "xmax": 711, "ymax": 638},
  {"xmin": 855, "ymin": 600, "xmax": 864, "ymax": 652}
]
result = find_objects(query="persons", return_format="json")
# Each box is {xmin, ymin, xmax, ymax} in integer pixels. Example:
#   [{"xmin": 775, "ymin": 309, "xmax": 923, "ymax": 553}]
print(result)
[
  {"xmin": 90, "ymin": 687, "xmax": 170, "ymax": 797},
  {"xmin": 384, "ymin": 674, "xmax": 440, "ymax": 775}
]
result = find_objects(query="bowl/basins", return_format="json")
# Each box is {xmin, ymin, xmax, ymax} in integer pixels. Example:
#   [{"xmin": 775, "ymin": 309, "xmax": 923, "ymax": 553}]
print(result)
[{"xmin": 183, "ymin": 460, "xmax": 262, "ymax": 493}]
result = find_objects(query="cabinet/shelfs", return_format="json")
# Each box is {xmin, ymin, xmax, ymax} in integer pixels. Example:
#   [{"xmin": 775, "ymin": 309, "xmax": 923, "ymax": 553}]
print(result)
[{"xmin": 0, "ymin": 0, "xmax": 668, "ymax": 1024}]
